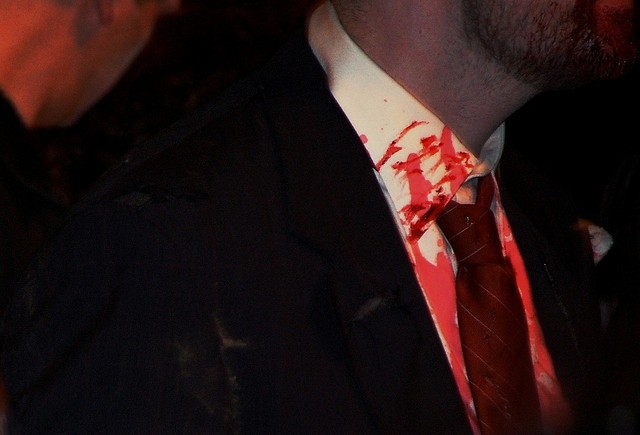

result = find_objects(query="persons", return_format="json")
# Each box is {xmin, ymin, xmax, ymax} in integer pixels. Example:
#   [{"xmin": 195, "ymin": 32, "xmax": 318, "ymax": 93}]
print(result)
[
  {"xmin": 0, "ymin": 0, "xmax": 184, "ymax": 377},
  {"xmin": 0, "ymin": 2, "xmax": 639, "ymax": 435}
]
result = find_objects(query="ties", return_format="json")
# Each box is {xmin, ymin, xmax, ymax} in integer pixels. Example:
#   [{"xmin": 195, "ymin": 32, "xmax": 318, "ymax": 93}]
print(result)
[{"xmin": 436, "ymin": 173, "xmax": 544, "ymax": 434}]
